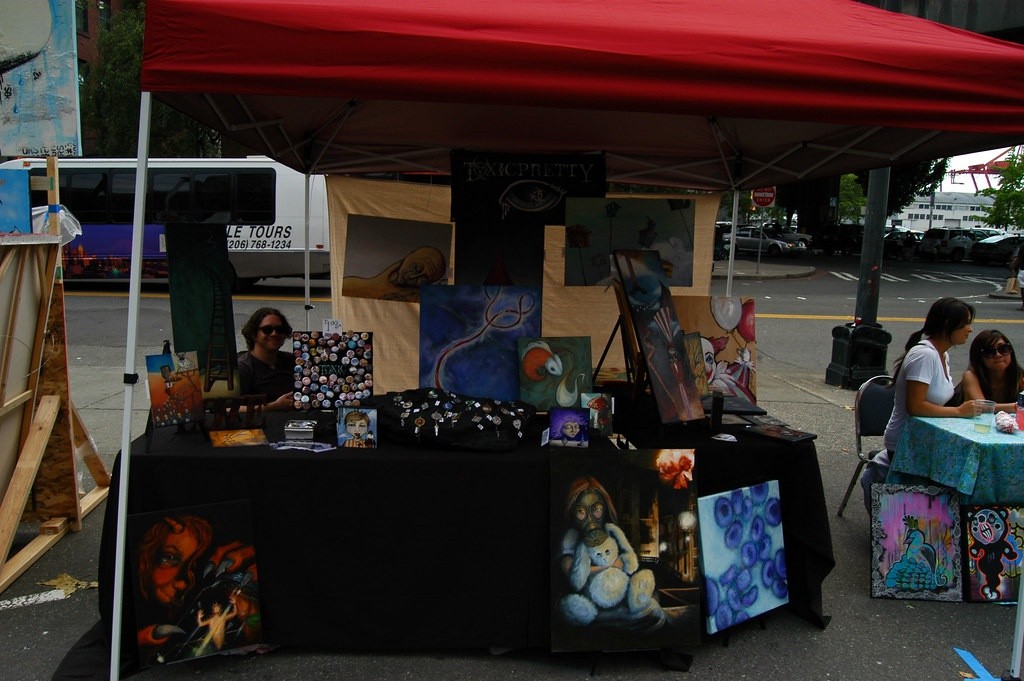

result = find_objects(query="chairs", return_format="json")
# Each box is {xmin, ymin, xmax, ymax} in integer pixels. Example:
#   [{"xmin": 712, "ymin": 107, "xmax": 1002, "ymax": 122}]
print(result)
[{"xmin": 837, "ymin": 375, "xmax": 895, "ymax": 518}]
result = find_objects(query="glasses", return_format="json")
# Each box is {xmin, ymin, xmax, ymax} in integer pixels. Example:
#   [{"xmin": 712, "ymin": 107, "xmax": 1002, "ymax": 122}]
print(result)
[
  {"xmin": 259, "ymin": 324, "xmax": 286, "ymax": 334},
  {"xmin": 983, "ymin": 342, "xmax": 1012, "ymax": 359}
]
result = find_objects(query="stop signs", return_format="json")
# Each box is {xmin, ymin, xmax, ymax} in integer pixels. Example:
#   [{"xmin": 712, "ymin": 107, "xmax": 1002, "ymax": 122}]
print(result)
[{"xmin": 749, "ymin": 186, "xmax": 776, "ymax": 208}]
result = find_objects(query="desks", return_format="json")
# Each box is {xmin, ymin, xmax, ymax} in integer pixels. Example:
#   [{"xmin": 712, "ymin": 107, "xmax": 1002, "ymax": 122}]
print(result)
[
  {"xmin": 885, "ymin": 413, "xmax": 1024, "ymax": 508},
  {"xmin": 53, "ymin": 395, "xmax": 837, "ymax": 681},
  {"xmin": 603, "ymin": 381, "xmax": 767, "ymax": 425}
]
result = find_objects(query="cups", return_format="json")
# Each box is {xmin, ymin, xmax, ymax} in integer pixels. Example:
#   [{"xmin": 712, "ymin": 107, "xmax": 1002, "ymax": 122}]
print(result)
[{"xmin": 973, "ymin": 399, "xmax": 996, "ymax": 433}]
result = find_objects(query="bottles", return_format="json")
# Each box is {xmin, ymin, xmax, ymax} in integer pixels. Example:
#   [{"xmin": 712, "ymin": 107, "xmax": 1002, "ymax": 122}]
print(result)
[{"xmin": 1016, "ymin": 391, "xmax": 1024, "ymax": 431}]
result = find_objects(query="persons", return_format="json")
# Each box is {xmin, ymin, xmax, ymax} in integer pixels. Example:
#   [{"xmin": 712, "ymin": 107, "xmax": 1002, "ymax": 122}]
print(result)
[
  {"xmin": 962, "ymin": 330, "xmax": 1024, "ymax": 414},
  {"xmin": 883, "ymin": 297, "xmax": 981, "ymax": 463},
  {"xmin": 237, "ymin": 306, "xmax": 295, "ymax": 411}
]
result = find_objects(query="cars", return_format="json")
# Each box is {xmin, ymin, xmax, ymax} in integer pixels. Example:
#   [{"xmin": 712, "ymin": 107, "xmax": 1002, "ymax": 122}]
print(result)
[
  {"xmin": 883, "ymin": 223, "xmax": 1024, "ymax": 273},
  {"xmin": 714, "ymin": 219, "xmax": 864, "ymax": 261}
]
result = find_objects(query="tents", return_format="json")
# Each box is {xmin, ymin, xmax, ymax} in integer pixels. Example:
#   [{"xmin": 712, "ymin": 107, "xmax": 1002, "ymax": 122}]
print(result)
[{"xmin": 110, "ymin": 0, "xmax": 1024, "ymax": 681}]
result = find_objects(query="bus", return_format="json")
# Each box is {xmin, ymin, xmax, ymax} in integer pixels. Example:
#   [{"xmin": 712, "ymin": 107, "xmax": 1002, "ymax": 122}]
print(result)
[{"xmin": 0, "ymin": 152, "xmax": 331, "ymax": 294}]
